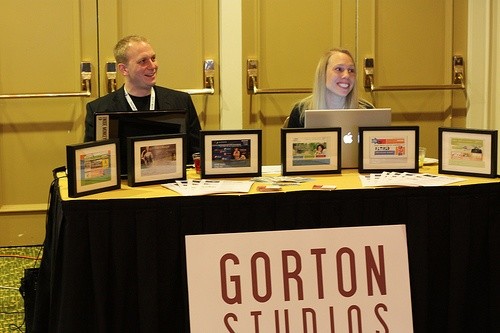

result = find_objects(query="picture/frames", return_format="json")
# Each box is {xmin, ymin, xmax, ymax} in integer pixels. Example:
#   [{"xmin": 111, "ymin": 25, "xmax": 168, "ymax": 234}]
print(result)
[
  {"xmin": 438, "ymin": 127, "xmax": 498, "ymax": 179},
  {"xmin": 200, "ymin": 129, "xmax": 262, "ymax": 179},
  {"xmin": 281, "ymin": 127, "xmax": 341, "ymax": 176},
  {"xmin": 358, "ymin": 126, "xmax": 419, "ymax": 173},
  {"xmin": 127, "ymin": 134, "xmax": 186, "ymax": 187},
  {"xmin": 66, "ymin": 139, "xmax": 121, "ymax": 198}
]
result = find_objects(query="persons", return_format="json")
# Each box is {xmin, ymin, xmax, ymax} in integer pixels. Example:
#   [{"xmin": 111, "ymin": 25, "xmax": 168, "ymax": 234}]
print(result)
[
  {"xmin": 233, "ymin": 148, "xmax": 247, "ymax": 160},
  {"xmin": 287, "ymin": 48, "xmax": 376, "ymax": 129},
  {"xmin": 314, "ymin": 145, "xmax": 327, "ymax": 156},
  {"xmin": 84, "ymin": 35, "xmax": 202, "ymax": 174}
]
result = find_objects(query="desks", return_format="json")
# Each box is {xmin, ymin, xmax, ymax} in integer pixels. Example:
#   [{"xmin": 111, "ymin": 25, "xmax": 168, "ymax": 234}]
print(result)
[{"xmin": 31, "ymin": 164, "xmax": 500, "ymax": 333}]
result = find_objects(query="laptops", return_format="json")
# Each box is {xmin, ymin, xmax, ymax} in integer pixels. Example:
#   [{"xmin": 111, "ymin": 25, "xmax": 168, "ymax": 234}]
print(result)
[
  {"xmin": 91, "ymin": 108, "xmax": 188, "ymax": 180},
  {"xmin": 304, "ymin": 108, "xmax": 392, "ymax": 169}
]
